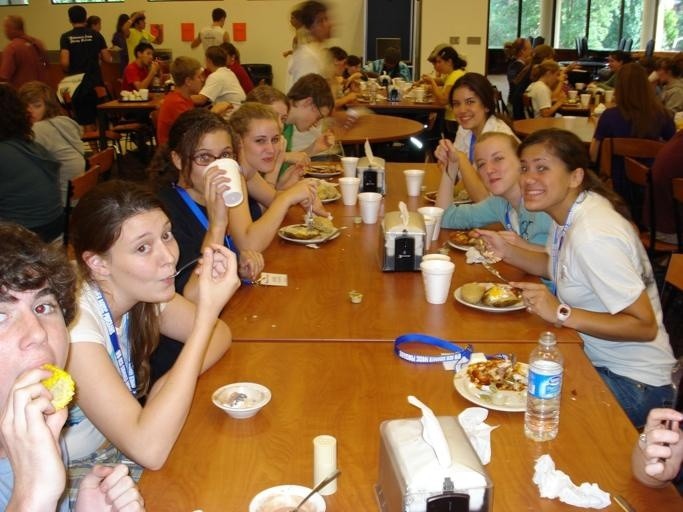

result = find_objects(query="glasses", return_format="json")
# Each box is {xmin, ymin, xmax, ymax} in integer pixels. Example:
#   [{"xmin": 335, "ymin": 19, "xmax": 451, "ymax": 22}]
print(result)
[{"xmin": 182, "ymin": 151, "xmax": 237, "ymax": 166}]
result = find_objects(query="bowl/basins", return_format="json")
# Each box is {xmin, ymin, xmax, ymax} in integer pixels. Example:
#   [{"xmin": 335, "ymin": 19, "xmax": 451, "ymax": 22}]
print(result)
[{"xmin": 210, "ymin": 381, "xmax": 272, "ymax": 419}]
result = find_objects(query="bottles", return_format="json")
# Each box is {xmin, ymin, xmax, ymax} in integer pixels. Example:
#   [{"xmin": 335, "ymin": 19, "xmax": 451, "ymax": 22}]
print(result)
[{"xmin": 521, "ymin": 327, "xmax": 565, "ymax": 444}]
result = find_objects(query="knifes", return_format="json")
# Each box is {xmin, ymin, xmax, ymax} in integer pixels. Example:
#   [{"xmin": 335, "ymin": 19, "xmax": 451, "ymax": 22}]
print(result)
[
  {"xmin": 654, "ymin": 354, "xmax": 683, "ymax": 472},
  {"xmin": 481, "ymin": 259, "xmax": 513, "ymax": 288}
]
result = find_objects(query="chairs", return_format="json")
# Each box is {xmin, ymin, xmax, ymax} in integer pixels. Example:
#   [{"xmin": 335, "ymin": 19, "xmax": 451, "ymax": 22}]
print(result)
[
  {"xmin": 62, "ymin": 146, "xmax": 116, "ymax": 252},
  {"xmin": 79, "ymin": 85, "xmax": 165, "ymax": 157},
  {"xmin": 557, "ymin": 36, "xmax": 658, "ymax": 88},
  {"xmin": 491, "ymin": 84, "xmax": 511, "ymax": 121},
  {"xmin": 658, "ymin": 254, "xmax": 683, "ymax": 307},
  {"xmin": 520, "ymin": 93, "xmax": 535, "ymax": 120},
  {"xmin": 599, "ymin": 132, "xmax": 683, "ymax": 274}
]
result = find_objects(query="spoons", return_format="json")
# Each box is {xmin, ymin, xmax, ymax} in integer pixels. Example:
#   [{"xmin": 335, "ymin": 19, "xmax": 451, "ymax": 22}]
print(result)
[{"xmin": 167, "ymin": 249, "xmax": 221, "ymax": 281}]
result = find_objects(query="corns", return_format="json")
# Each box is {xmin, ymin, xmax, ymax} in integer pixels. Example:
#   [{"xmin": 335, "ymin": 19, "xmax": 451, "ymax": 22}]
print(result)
[{"xmin": 35, "ymin": 363, "xmax": 75, "ymax": 413}]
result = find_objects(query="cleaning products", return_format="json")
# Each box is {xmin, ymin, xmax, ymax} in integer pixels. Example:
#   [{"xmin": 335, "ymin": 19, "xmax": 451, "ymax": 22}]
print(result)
[
  {"xmin": 399, "ymin": 62, "xmax": 410, "ymax": 82},
  {"xmin": 367, "ymin": 59, "xmax": 385, "ymax": 72}
]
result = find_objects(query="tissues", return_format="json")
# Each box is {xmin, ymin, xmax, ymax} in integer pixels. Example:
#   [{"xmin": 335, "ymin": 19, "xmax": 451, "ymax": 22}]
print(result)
[
  {"xmin": 380, "ymin": 70, "xmax": 391, "ymax": 86},
  {"xmin": 374, "ymin": 395, "xmax": 494, "ymax": 512},
  {"xmin": 377, "ymin": 202, "xmax": 427, "ymax": 273},
  {"xmin": 386, "ymin": 79, "xmax": 401, "ymax": 101},
  {"xmin": 355, "ymin": 139, "xmax": 386, "ymax": 196}
]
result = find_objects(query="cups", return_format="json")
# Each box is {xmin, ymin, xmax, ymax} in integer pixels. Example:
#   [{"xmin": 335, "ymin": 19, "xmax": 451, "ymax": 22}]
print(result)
[
  {"xmin": 567, "ymin": 83, "xmax": 614, "ymax": 107},
  {"xmin": 202, "ymin": 158, "xmax": 244, "ymax": 209},
  {"xmin": 647, "ymin": 71, "xmax": 660, "ymax": 85},
  {"xmin": 139, "ymin": 88, "xmax": 149, "ymax": 100},
  {"xmin": 420, "ymin": 253, "xmax": 456, "ymax": 305},
  {"xmin": 338, "ymin": 156, "xmax": 445, "ymax": 251},
  {"xmin": 357, "ymin": 76, "xmax": 430, "ymax": 103}
]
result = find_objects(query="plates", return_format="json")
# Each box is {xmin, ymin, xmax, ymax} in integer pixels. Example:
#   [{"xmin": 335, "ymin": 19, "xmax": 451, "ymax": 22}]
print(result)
[
  {"xmin": 447, "ymin": 232, "xmax": 475, "ymax": 251},
  {"xmin": 248, "ymin": 484, "xmax": 327, "ymax": 512},
  {"xmin": 452, "ymin": 282, "xmax": 527, "ymax": 313},
  {"xmin": 303, "ymin": 164, "xmax": 342, "ymax": 178},
  {"xmin": 451, "ymin": 359, "xmax": 530, "ymax": 413},
  {"xmin": 277, "ymin": 223, "xmax": 340, "ymax": 244},
  {"xmin": 316, "ymin": 184, "xmax": 342, "ymax": 203}
]
